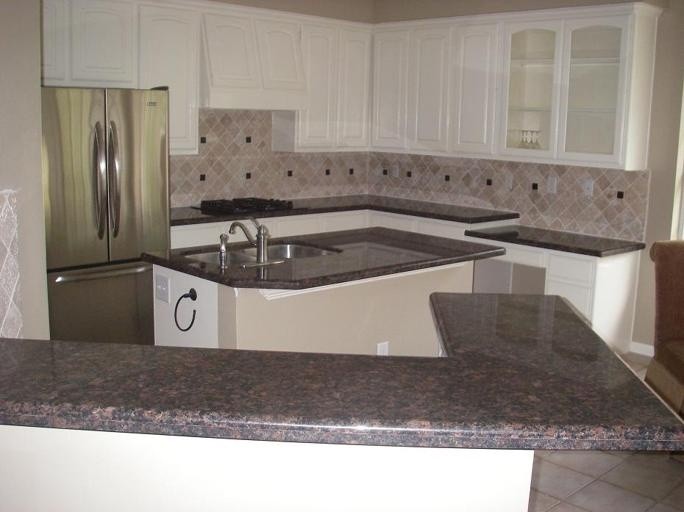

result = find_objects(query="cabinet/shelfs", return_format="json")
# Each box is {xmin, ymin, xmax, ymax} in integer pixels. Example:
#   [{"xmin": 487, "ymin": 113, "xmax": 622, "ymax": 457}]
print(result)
[
  {"xmin": 545, "ymin": 250, "xmax": 594, "ymax": 325},
  {"xmin": 376, "ymin": 23, "xmax": 411, "ymax": 153},
  {"xmin": 272, "ymin": 18, "xmax": 373, "ymax": 152},
  {"xmin": 496, "ymin": 4, "xmax": 663, "ymax": 173},
  {"xmin": 44, "ymin": 1, "xmax": 140, "ymax": 90},
  {"xmin": 412, "ymin": 11, "xmax": 502, "ymax": 159},
  {"xmin": 199, "ymin": 0, "xmax": 307, "ymax": 109},
  {"xmin": 138, "ymin": 3, "xmax": 199, "ymax": 155}
]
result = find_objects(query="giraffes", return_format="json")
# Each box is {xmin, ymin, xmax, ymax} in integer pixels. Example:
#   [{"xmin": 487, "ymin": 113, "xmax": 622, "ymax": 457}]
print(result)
[{"xmin": 191, "ymin": 196, "xmax": 294, "ymax": 217}]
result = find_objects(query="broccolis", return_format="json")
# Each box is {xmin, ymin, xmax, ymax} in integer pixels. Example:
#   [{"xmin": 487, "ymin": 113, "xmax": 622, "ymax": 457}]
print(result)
[{"xmin": 40, "ymin": 86, "xmax": 168, "ymax": 346}]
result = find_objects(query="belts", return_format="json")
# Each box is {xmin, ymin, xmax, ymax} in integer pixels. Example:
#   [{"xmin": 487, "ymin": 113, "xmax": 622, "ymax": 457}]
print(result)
[{"xmin": 516, "ymin": 128, "xmax": 544, "ymax": 151}]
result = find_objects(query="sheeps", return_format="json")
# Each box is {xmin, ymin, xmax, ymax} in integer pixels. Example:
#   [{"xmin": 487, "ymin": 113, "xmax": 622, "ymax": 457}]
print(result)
[
  {"xmin": 228, "ymin": 214, "xmax": 268, "ymax": 264},
  {"xmin": 240, "ymin": 265, "xmax": 269, "ymax": 282}
]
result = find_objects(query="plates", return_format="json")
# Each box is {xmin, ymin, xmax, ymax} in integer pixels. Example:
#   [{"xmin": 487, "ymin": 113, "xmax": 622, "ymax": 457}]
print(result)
[{"xmin": 545, "ymin": 250, "xmax": 594, "ymax": 325}]
